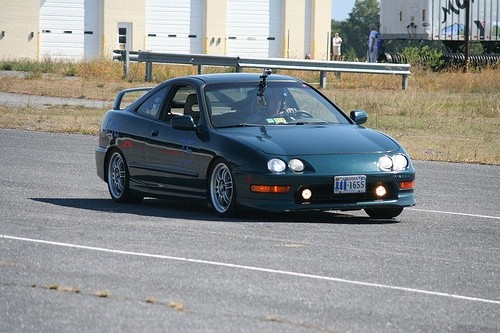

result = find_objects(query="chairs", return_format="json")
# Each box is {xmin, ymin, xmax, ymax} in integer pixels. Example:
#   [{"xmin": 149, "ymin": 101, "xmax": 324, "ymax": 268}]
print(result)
[{"xmin": 184, "ymin": 93, "xmax": 216, "ymax": 127}]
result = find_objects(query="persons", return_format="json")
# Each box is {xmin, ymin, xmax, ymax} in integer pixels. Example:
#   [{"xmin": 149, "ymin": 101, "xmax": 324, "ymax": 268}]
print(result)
[
  {"xmin": 332, "ymin": 33, "xmax": 343, "ymax": 61},
  {"xmin": 367, "ymin": 23, "xmax": 384, "ymax": 63},
  {"xmin": 257, "ymin": 95, "xmax": 297, "ymax": 123}
]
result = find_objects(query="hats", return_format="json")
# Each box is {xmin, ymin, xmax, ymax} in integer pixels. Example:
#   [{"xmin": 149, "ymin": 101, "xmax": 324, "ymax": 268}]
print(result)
[{"xmin": 368, "ymin": 23, "xmax": 376, "ymax": 30}]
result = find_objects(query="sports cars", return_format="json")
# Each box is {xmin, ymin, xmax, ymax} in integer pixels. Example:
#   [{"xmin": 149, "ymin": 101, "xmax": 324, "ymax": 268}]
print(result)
[{"xmin": 93, "ymin": 71, "xmax": 418, "ymax": 220}]
